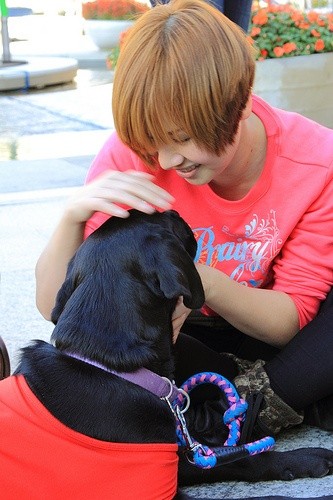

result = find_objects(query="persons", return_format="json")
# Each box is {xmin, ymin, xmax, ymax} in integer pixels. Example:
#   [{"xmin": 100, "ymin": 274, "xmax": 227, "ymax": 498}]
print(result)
[{"xmin": 36, "ymin": 0, "xmax": 333, "ymax": 428}]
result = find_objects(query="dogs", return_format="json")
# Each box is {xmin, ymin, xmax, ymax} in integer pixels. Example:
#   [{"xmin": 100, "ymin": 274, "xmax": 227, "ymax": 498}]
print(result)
[{"xmin": 0, "ymin": 208, "xmax": 333, "ymax": 498}]
